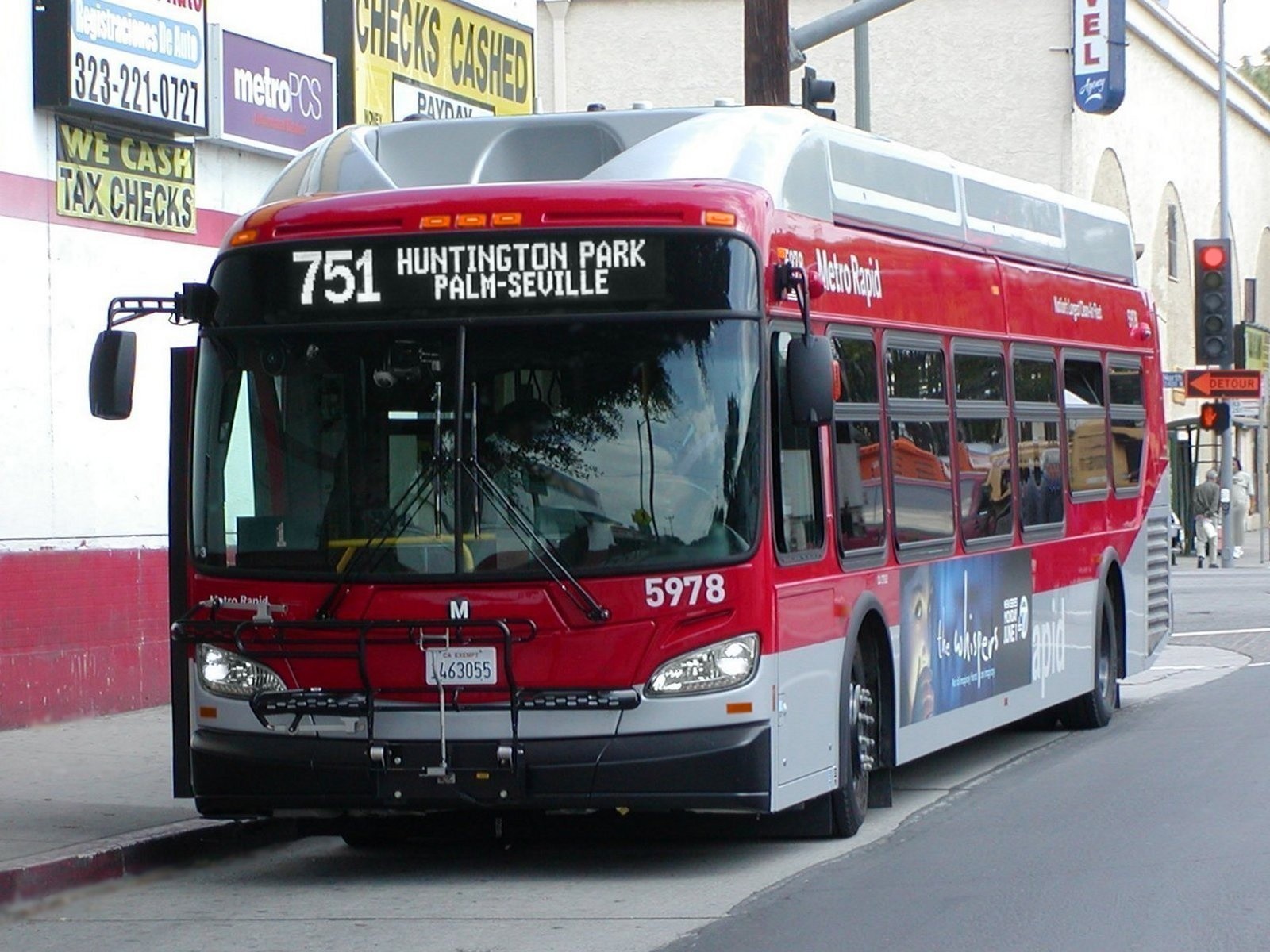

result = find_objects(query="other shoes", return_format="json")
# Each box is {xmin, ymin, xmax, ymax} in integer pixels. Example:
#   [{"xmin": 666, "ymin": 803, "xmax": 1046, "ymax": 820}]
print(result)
[
  {"xmin": 1233, "ymin": 547, "xmax": 1244, "ymax": 558},
  {"xmin": 1209, "ymin": 564, "xmax": 1218, "ymax": 568},
  {"xmin": 1198, "ymin": 558, "xmax": 1203, "ymax": 568}
]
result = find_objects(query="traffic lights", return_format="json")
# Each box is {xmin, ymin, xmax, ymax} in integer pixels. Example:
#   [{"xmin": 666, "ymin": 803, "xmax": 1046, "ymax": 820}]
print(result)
[
  {"xmin": 802, "ymin": 69, "xmax": 837, "ymax": 120},
  {"xmin": 1195, "ymin": 238, "xmax": 1235, "ymax": 364},
  {"xmin": 1201, "ymin": 402, "xmax": 1231, "ymax": 434}
]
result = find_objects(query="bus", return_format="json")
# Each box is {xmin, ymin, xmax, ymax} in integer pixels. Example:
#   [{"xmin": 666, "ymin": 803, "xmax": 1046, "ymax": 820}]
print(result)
[{"xmin": 89, "ymin": 99, "xmax": 1185, "ymax": 844}]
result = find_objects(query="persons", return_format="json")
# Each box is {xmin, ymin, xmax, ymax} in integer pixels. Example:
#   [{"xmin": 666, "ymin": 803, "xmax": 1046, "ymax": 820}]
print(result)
[
  {"xmin": 1191, "ymin": 469, "xmax": 1220, "ymax": 568},
  {"xmin": 320, "ymin": 340, "xmax": 735, "ymax": 550},
  {"xmin": 1231, "ymin": 457, "xmax": 1256, "ymax": 559}
]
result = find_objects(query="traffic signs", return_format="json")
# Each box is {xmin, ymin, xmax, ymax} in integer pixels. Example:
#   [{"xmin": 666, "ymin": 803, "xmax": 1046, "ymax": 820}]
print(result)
[{"xmin": 1186, "ymin": 370, "xmax": 1261, "ymax": 398}]
